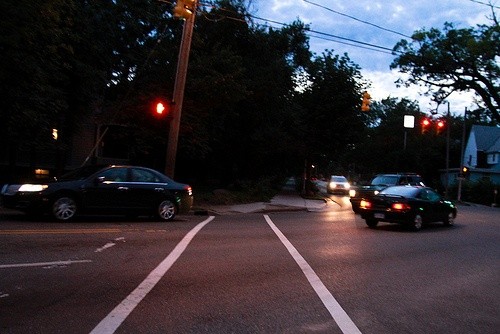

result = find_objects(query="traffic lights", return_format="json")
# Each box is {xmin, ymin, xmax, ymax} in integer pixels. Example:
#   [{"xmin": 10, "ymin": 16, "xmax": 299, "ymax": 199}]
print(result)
[
  {"xmin": 423, "ymin": 119, "xmax": 444, "ymax": 127},
  {"xmin": 156, "ymin": 101, "xmax": 165, "ymax": 113},
  {"xmin": 462, "ymin": 166, "xmax": 468, "ymax": 178}
]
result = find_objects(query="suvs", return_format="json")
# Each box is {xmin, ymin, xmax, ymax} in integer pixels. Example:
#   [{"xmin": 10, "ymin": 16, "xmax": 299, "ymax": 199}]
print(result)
[
  {"xmin": 348, "ymin": 173, "xmax": 422, "ymax": 215},
  {"xmin": 327, "ymin": 175, "xmax": 350, "ymax": 195}
]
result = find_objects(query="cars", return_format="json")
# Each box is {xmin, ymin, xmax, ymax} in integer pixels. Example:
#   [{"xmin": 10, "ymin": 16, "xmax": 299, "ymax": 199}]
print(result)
[
  {"xmin": 360, "ymin": 184, "xmax": 457, "ymax": 231},
  {"xmin": 0, "ymin": 164, "xmax": 194, "ymax": 222}
]
